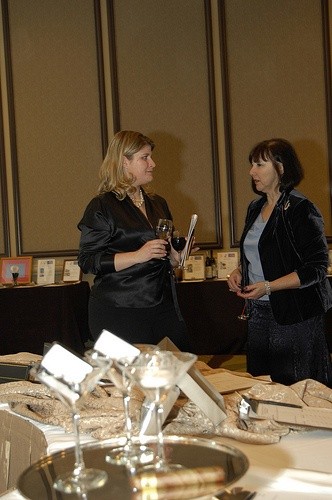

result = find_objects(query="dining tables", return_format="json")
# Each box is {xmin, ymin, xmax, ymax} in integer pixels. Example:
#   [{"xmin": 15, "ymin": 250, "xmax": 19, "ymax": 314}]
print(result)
[{"xmin": 1, "ymin": 351, "xmax": 331, "ymax": 500}]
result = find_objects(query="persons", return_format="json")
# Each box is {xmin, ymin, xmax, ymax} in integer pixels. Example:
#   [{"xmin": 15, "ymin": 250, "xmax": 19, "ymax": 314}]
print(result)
[
  {"xmin": 226, "ymin": 137, "xmax": 332, "ymax": 390},
  {"xmin": 76, "ymin": 129, "xmax": 200, "ymax": 356}
]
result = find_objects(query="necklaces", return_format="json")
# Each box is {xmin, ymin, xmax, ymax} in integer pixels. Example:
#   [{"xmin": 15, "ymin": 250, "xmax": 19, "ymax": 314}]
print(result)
[{"xmin": 127, "ymin": 185, "xmax": 143, "ymax": 207}]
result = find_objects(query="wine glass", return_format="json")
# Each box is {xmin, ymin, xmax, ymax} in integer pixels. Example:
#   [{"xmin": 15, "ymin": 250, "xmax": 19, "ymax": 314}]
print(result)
[
  {"xmin": 171, "ymin": 231, "xmax": 188, "ymax": 270},
  {"xmin": 155, "ymin": 219, "xmax": 174, "ymax": 261},
  {"xmin": 85, "ymin": 345, "xmax": 159, "ymax": 466},
  {"xmin": 121, "ymin": 352, "xmax": 197, "ymax": 469},
  {"xmin": 28, "ymin": 356, "xmax": 108, "ymax": 490}
]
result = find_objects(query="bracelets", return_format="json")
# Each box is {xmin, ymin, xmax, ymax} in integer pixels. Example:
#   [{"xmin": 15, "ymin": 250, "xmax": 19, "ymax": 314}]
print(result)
[{"xmin": 265, "ymin": 280, "xmax": 271, "ymax": 295}]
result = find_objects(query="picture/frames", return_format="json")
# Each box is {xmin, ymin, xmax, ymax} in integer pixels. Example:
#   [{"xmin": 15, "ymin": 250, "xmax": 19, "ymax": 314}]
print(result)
[{"xmin": 0, "ymin": 256, "xmax": 33, "ymax": 285}]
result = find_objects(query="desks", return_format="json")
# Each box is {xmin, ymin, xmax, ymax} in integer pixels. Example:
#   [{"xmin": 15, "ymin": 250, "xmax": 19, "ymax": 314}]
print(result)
[
  {"xmin": 175, "ymin": 274, "xmax": 332, "ymax": 354},
  {"xmin": 0, "ymin": 280, "xmax": 90, "ymax": 355}
]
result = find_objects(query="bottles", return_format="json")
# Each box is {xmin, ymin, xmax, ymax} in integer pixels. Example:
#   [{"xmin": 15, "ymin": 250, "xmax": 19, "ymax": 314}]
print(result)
[{"xmin": 206, "ymin": 249, "xmax": 216, "ymax": 279}]
